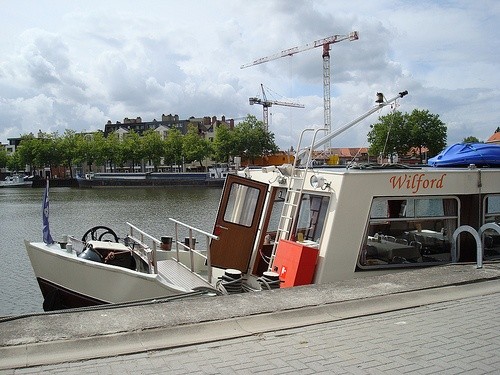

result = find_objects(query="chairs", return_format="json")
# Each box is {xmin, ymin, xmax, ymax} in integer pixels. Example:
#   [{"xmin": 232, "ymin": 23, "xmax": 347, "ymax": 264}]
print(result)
[{"xmin": 363, "ymin": 226, "xmax": 455, "ymax": 264}]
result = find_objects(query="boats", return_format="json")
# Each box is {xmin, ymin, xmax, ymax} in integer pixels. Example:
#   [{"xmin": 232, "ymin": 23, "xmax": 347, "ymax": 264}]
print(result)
[
  {"xmin": 75, "ymin": 163, "xmax": 236, "ymax": 188},
  {"xmin": 0, "ymin": 170, "xmax": 35, "ymax": 188},
  {"xmin": 22, "ymin": 89, "xmax": 500, "ymax": 312}
]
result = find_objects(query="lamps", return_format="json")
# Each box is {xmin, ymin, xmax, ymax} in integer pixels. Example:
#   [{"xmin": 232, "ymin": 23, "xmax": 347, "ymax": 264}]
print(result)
[
  {"xmin": 312, "ymin": 174, "xmax": 331, "ymax": 190},
  {"xmin": 279, "ymin": 165, "xmax": 288, "ymax": 178}
]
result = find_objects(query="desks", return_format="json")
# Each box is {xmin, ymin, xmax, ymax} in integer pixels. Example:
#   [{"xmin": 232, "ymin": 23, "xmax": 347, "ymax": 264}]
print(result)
[
  {"xmin": 367, "ymin": 236, "xmax": 420, "ymax": 260},
  {"xmin": 485, "ymin": 231, "xmax": 499, "ymax": 255},
  {"xmin": 409, "ymin": 230, "xmax": 442, "ymax": 255}
]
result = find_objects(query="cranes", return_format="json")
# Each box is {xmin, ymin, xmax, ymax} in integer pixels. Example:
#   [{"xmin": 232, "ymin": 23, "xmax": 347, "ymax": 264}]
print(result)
[
  {"xmin": 248, "ymin": 83, "xmax": 306, "ymax": 144},
  {"xmin": 240, "ymin": 31, "xmax": 360, "ymax": 155}
]
